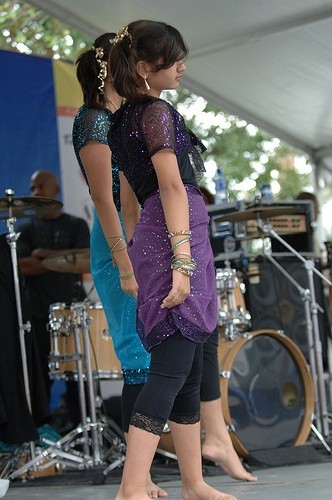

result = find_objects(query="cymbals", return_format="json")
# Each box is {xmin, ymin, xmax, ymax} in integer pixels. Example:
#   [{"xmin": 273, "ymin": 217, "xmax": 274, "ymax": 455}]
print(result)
[
  {"xmin": 41, "ymin": 248, "xmax": 91, "ymax": 274},
  {"xmin": 0, "ymin": 196, "xmax": 64, "ymax": 220},
  {"xmin": 214, "ymin": 206, "xmax": 300, "ymax": 224}
]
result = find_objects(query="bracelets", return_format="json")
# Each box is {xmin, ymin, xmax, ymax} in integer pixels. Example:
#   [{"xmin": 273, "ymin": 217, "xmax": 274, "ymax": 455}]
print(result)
[
  {"xmin": 105, "ymin": 234, "xmax": 134, "ymax": 279},
  {"xmin": 167, "ymin": 230, "xmax": 197, "ymax": 276}
]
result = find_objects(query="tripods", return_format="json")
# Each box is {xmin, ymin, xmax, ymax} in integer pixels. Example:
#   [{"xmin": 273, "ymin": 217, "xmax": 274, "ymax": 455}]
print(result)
[{"xmin": 0, "ymin": 190, "xmax": 206, "ymax": 484}]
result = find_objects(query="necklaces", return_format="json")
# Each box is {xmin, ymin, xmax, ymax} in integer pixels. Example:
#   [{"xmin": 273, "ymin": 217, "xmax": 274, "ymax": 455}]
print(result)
[{"xmin": 107, "ymin": 96, "xmax": 118, "ymax": 111}]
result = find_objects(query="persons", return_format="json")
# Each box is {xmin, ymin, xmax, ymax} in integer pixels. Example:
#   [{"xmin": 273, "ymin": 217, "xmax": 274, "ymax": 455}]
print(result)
[
  {"xmin": 15, "ymin": 169, "xmax": 92, "ymax": 456},
  {"xmin": 106, "ymin": 19, "xmax": 238, "ymax": 500},
  {"xmin": 293, "ymin": 192, "xmax": 320, "ymax": 222},
  {"xmin": 70, "ymin": 33, "xmax": 258, "ymax": 498}
]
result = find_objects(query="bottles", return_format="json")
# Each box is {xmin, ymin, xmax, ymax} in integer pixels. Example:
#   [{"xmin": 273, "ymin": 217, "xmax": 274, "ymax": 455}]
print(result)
[
  {"xmin": 215, "ymin": 168, "xmax": 228, "ymax": 204},
  {"xmin": 259, "ymin": 183, "xmax": 274, "ymax": 203}
]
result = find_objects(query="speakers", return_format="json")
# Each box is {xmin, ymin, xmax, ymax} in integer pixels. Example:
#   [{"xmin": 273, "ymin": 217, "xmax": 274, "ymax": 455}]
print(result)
[{"xmin": 239, "ymin": 261, "xmax": 328, "ymax": 373}]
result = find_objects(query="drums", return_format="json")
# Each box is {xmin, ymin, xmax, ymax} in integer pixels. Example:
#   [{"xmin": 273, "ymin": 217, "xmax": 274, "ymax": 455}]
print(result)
[
  {"xmin": 48, "ymin": 303, "xmax": 126, "ymax": 380},
  {"xmin": 122, "ymin": 329, "xmax": 316, "ymax": 459},
  {"xmin": 214, "ymin": 269, "xmax": 252, "ymax": 331}
]
